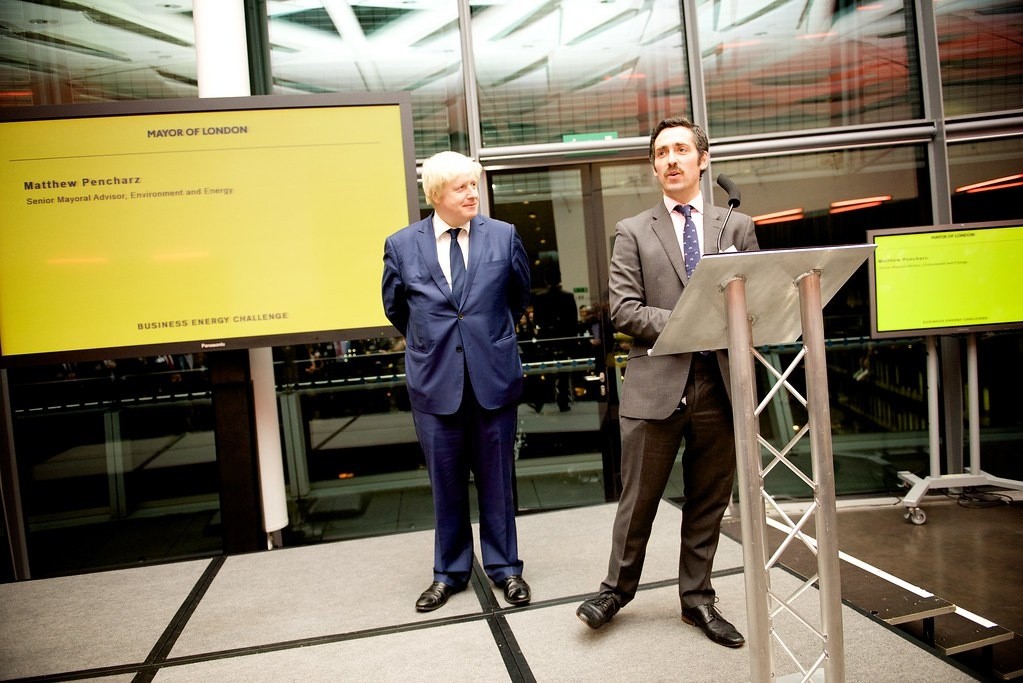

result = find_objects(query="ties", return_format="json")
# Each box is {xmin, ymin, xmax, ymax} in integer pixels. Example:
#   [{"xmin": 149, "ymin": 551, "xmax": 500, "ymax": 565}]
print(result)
[
  {"xmin": 673, "ymin": 205, "xmax": 700, "ymax": 279},
  {"xmin": 445, "ymin": 228, "xmax": 467, "ymax": 307}
]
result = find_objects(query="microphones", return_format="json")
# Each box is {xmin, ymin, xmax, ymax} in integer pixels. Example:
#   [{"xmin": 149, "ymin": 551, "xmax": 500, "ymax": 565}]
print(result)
[{"xmin": 716, "ymin": 174, "xmax": 741, "ymax": 252}]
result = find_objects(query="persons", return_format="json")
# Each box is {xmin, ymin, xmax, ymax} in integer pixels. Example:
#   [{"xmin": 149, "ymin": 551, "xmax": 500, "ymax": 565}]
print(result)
[
  {"xmin": 51, "ymin": 303, "xmax": 612, "ymax": 402},
  {"xmin": 383, "ymin": 149, "xmax": 531, "ymax": 614},
  {"xmin": 526, "ymin": 265, "xmax": 577, "ymax": 413},
  {"xmin": 576, "ymin": 117, "xmax": 744, "ymax": 648}
]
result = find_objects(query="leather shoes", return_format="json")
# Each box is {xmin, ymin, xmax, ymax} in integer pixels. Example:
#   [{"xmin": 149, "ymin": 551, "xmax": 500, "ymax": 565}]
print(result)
[
  {"xmin": 680, "ymin": 595, "xmax": 745, "ymax": 647},
  {"xmin": 414, "ymin": 580, "xmax": 468, "ymax": 610},
  {"xmin": 575, "ymin": 595, "xmax": 620, "ymax": 629},
  {"xmin": 489, "ymin": 573, "xmax": 532, "ymax": 604}
]
